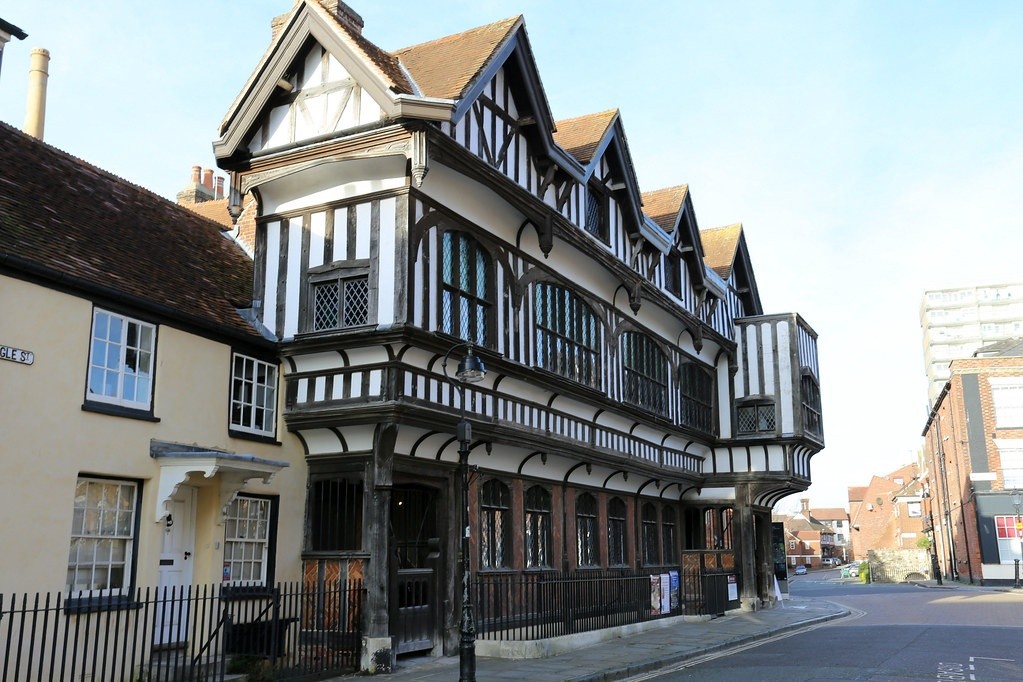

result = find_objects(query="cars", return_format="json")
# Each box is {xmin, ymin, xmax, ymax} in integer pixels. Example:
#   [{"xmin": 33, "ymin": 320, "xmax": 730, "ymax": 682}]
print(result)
[
  {"xmin": 793, "ymin": 565, "xmax": 807, "ymax": 575},
  {"xmin": 822, "ymin": 557, "xmax": 867, "ymax": 578}
]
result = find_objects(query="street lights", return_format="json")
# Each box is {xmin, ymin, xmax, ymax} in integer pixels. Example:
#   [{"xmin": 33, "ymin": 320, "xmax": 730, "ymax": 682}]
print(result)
[
  {"xmin": 442, "ymin": 330, "xmax": 489, "ymax": 682},
  {"xmin": 1009, "ymin": 485, "xmax": 1023, "ymax": 571}
]
